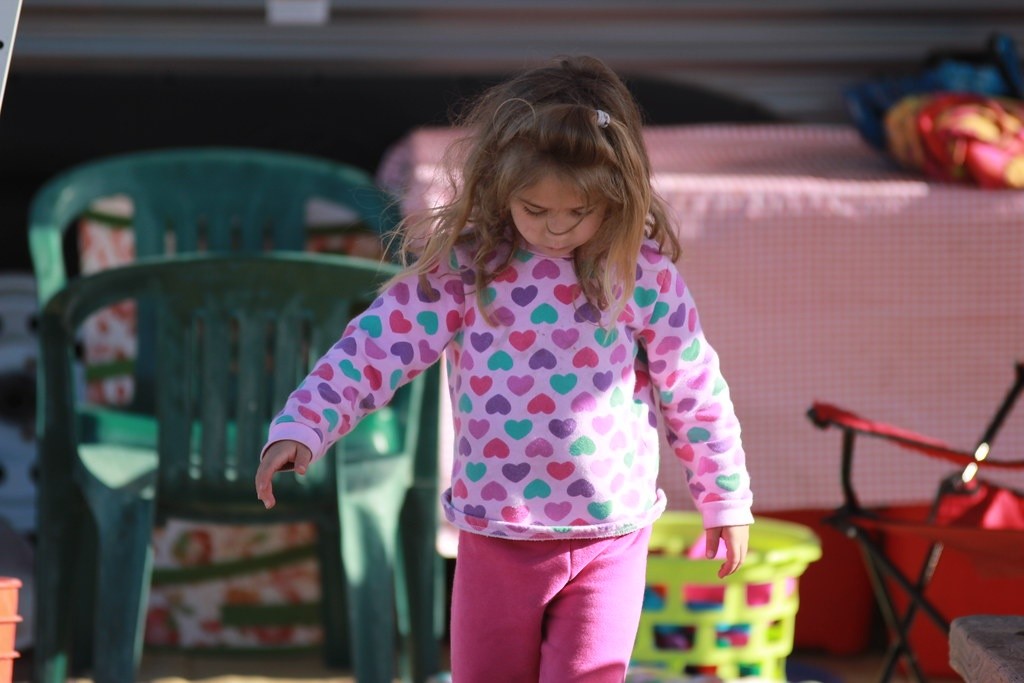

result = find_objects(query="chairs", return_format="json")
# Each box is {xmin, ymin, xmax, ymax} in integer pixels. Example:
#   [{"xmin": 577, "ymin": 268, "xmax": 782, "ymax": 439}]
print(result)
[
  {"xmin": 27, "ymin": 148, "xmax": 437, "ymax": 683},
  {"xmin": 807, "ymin": 361, "xmax": 1024, "ymax": 683},
  {"xmin": 31, "ymin": 251, "xmax": 441, "ymax": 683}
]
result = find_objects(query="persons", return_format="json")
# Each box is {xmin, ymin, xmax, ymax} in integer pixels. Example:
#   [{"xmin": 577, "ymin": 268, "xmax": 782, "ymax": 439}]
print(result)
[{"xmin": 254, "ymin": 53, "xmax": 756, "ymax": 683}]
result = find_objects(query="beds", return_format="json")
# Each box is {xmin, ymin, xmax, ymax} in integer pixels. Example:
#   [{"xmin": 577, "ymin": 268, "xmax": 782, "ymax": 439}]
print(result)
[{"xmin": 368, "ymin": 34, "xmax": 1024, "ymax": 654}]
